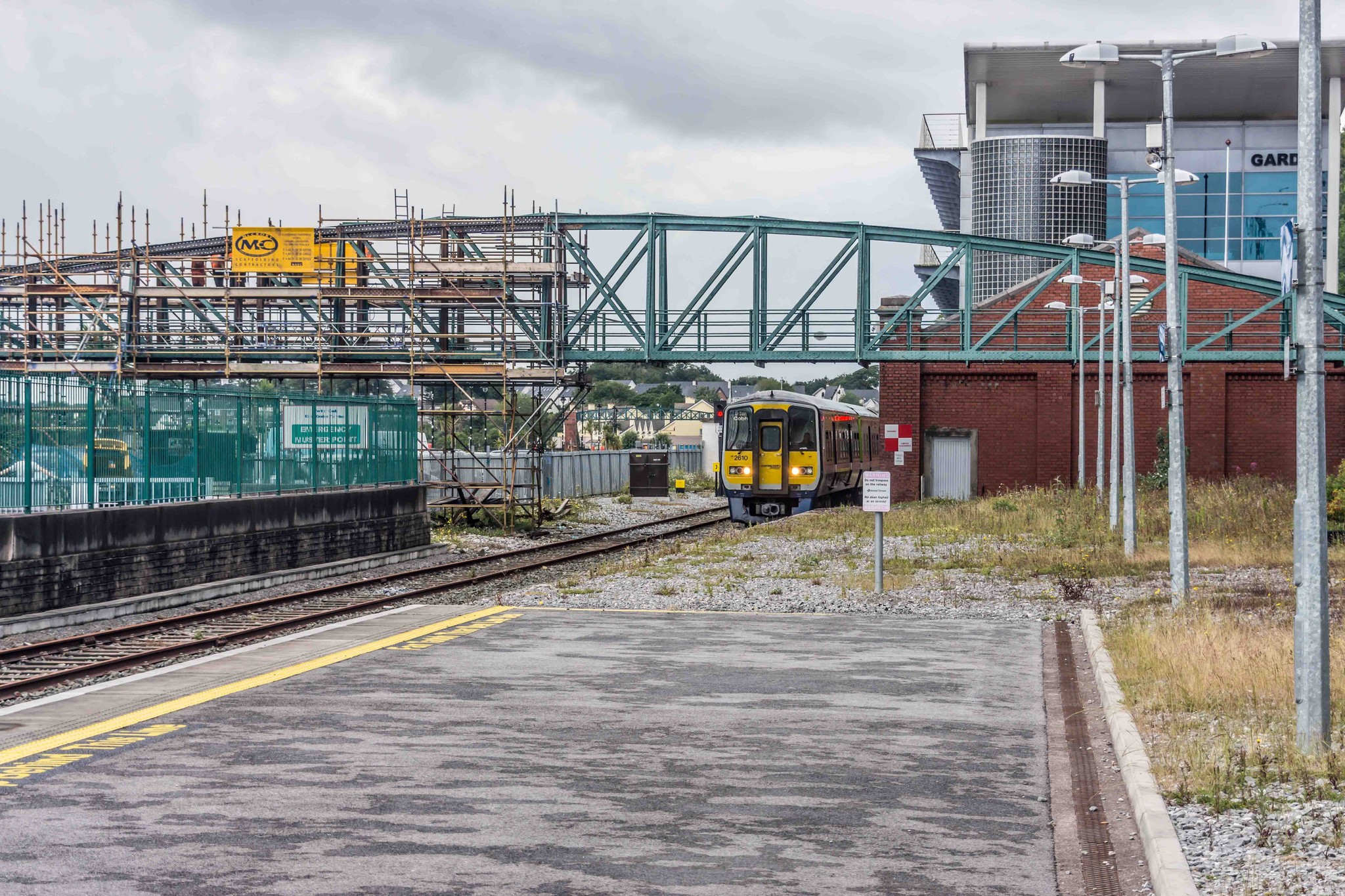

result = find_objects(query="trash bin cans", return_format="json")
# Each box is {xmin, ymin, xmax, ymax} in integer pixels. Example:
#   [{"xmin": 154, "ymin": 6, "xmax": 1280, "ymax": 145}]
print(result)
[{"xmin": 629, "ymin": 448, "xmax": 671, "ymax": 497}]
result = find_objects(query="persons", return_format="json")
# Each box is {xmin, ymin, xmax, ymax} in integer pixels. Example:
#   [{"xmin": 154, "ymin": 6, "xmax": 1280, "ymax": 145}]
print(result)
[{"xmin": 190, "ymin": 253, "xmax": 247, "ymax": 287}]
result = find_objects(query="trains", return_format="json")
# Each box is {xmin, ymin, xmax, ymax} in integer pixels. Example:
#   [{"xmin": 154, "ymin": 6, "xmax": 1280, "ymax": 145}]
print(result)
[{"xmin": 721, "ymin": 389, "xmax": 881, "ymax": 529}]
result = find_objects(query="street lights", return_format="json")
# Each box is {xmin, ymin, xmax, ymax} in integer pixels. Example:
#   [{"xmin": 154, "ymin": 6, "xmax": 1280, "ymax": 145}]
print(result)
[
  {"xmin": 1058, "ymin": 36, "xmax": 1278, "ymax": 621},
  {"xmin": 1042, "ymin": 301, "xmax": 1117, "ymax": 497},
  {"xmin": 1048, "ymin": 168, "xmax": 1202, "ymax": 556},
  {"xmin": 1056, "ymin": 275, "xmax": 1152, "ymax": 510}
]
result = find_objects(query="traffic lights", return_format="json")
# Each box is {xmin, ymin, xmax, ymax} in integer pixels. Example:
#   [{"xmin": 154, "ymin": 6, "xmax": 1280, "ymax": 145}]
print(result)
[{"xmin": 713, "ymin": 400, "xmax": 727, "ymax": 423}]
